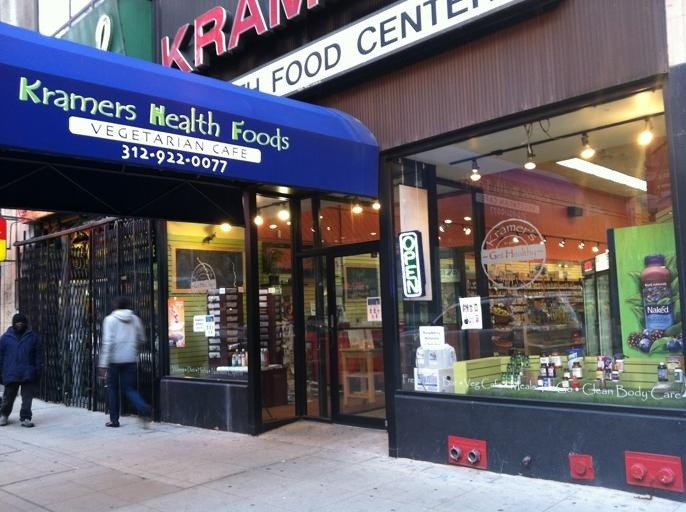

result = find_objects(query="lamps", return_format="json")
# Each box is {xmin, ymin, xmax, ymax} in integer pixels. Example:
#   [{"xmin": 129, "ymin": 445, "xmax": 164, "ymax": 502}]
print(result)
[
  {"xmin": 438, "ymin": 220, "xmax": 600, "ymax": 254},
  {"xmin": 464, "ymin": 132, "xmax": 597, "ymax": 183}
]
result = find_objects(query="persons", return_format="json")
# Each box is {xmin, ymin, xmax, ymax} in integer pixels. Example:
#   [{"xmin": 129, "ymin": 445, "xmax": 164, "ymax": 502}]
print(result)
[
  {"xmin": 0, "ymin": 313, "xmax": 43, "ymax": 427},
  {"xmin": 96, "ymin": 296, "xmax": 153, "ymax": 429}
]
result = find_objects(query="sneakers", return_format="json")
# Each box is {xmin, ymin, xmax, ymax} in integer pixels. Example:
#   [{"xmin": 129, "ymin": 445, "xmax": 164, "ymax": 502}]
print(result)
[
  {"xmin": 21, "ymin": 419, "xmax": 34, "ymax": 427},
  {"xmin": 0, "ymin": 415, "xmax": 8, "ymax": 427},
  {"xmin": 106, "ymin": 421, "xmax": 120, "ymax": 427}
]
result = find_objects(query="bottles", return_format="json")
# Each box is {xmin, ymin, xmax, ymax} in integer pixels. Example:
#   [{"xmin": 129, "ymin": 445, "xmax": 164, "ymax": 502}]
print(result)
[
  {"xmin": 658, "ymin": 361, "xmax": 683, "ymax": 384},
  {"xmin": 502, "ymin": 351, "xmax": 530, "ymax": 386},
  {"xmin": 596, "ymin": 353, "xmax": 624, "ymax": 388},
  {"xmin": 231, "ymin": 348, "xmax": 270, "ymax": 367},
  {"xmin": 640, "ymin": 255, "xmax": 675, "ymax": 331},
  {"xmin": 537, "ymin": 351, "xmax": 583, "ymax": 388}
]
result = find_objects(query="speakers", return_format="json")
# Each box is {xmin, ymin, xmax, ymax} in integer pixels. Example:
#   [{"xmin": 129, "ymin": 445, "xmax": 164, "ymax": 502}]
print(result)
[{"xmin": 568, "ymin": 206, "xmax": 583, "ymax": 217}]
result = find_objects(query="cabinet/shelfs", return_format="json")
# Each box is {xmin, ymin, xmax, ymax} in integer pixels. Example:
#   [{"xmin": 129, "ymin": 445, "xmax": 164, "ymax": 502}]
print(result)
[
  {"xmin": 465, "ymin": 276, "xmax": 584, "ymax": 313},
  {"xmin": 206, "ymin": 284, "xmax": 288, "ymax": 407},
  {"xmin": 338, "ymin": 341, "xmax": 384, "ymax": 407},
  {"xmin": 511, "ymin": 326, "xmax": 584, "ymax": 355}
]
